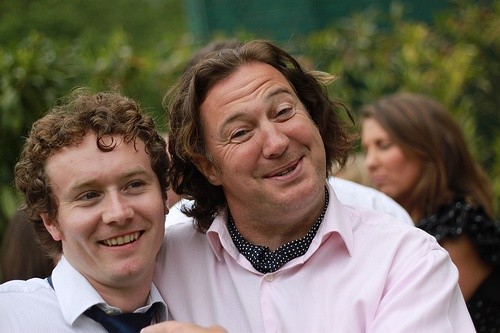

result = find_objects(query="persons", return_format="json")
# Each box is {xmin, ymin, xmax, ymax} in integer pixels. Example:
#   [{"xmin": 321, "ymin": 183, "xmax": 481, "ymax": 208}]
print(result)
[
  {"xmin": 140, "ymin": 39, "xmax": 477, "ymax": 333},
  {"xmin": 360, "ymin": 94, "xmax": 500, "ymax": 333},
  {"xmin": 0, "ymin": 69, "xmax": 170, "ymax": 333}
]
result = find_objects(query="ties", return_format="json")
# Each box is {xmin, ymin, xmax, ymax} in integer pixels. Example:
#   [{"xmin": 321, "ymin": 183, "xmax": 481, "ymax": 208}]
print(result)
[{"xmin": 47, "ymin": 276, "xmax": 160, "ymax": 333}]
